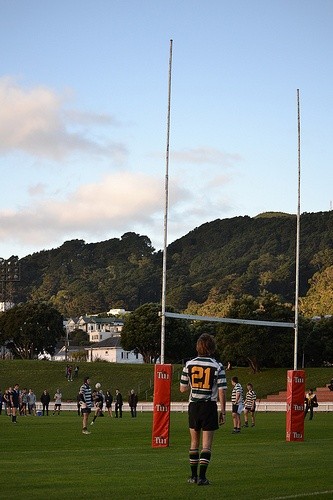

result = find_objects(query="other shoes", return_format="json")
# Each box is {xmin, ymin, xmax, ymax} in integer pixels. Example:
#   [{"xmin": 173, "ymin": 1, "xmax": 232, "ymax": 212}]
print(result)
[
  {"xmin": 81, "ymin": 428, "xmax": 92, "ymax": 434},
  {"xmin": 11, "ymin": 421, "xmax": 17, "ymax": 424},
  {"xmin": 90, "ymin": 421, "xmax": 94, "ymax": 426},
  {"xmin": 251, "ymin": 425, "xmax": 256, "ymax": 428},
  {"xmin": 186, "ymin": 476, "xmax": 197, "ymax": 484},
  {"xmin": 231, "ymin": 429, "xmax": 241, "ymax": 434},
  {"xmin": 197, "ymin": 478, "xmax": 210, "ymax": 486},
  {"xmin": 241, "ymin": 424, "xmax": 249, "ymax": 428}
]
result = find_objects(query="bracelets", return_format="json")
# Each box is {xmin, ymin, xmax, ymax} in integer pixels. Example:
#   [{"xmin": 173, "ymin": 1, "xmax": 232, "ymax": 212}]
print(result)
[{"xmin": 222, "ymin": 413, "xmax": 225, "ymax": 415}]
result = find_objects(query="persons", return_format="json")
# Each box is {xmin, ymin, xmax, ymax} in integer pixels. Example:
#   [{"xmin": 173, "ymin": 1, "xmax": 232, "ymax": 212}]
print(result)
[
  {"xmin": 114, "ymin": 389, "xmax": 123, "ymax": 418},
  {"xmin": 128, "ymin": 389, "xmax": 138, "ymax": 417},
  {"xmin": 328, "ymin": 377, "xmax": 333, "ymax": 391},
  {"xmin": 243, "ymin": 383, "xmax": 257, "ymax": 428},
  {"xmin": 65, "ymin": 364, "xmax": 79, "ymax": 382},
  {"xmin": 180, "ymin": 334, "xmax": 228, "ymax": 485},
  {"xmin": 79, "ymin": 377, "xmax": 93, "ymax": 434},
  {"xmin": 40, "ymin": 389, "xmax": 50, "ymax": 416},
  {"xmin": 105, "ymin": 391, "xmax": 113, "ymax": 417},
  {"xmin": 53, "ymin": 389, "xmax": 62, "ymax": 416},
  {"xmin": 76, "ymin": 391, "xmax": 83, "ymax": 416},
  {"xmin": 231, "ymin": 376, "xmax": 244, "ymax": 434},
  {"xmin": 304, "ymin": 388, "xmax": 317, "ymax": 420},
  {"xmin": 226, "ymin": 362, "xmax": 230, "ymax": 370},
  {"xmin": 90, "ymin": 390, "xmax": 105, "ymax": 425},
  {"xmin": 0, "ymin": 384, "xmax": 36, "ymax": 423}
]
